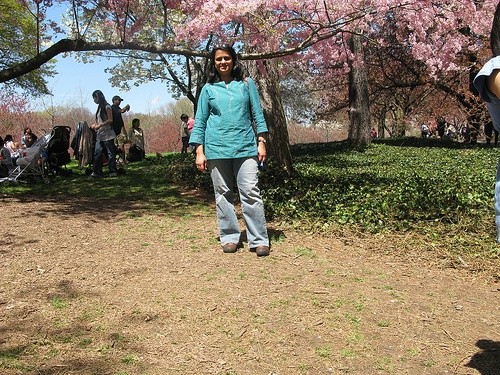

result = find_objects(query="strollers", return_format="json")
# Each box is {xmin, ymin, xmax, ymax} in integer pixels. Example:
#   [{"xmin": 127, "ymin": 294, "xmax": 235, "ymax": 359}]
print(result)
[
  {"xmin": 75, "ymin": 120, "xmax": 123, "ymax": 174},
  {"xmin": 0, "ymin": 127, "xmax": 55, "ymax": 186},
  {"xmin": 45, "ymin": 125, "xmax": 73, "ymax": 175}
]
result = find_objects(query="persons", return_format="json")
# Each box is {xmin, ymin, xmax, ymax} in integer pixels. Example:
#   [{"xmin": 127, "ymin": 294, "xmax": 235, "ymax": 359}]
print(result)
[
  {"xmin": 0, "ymin": 91, "xmax": 197, "ymax": 178},
  {"xmin": 189, "ymin": 45, "xmax": 271, "ymax": 257},
  {"xmin": 472, "ymin": 0, "xmax": 500, "ymax": 245},
  {"xmin": 368, "ymin": 114, "xmax": 498, "ymax": 146}
]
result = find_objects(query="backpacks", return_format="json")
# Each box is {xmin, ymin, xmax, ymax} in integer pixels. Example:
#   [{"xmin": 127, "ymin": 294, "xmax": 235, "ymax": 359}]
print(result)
[{"xmin": 126, "ymin": 145, "xmax": 145, "ymax": 162}]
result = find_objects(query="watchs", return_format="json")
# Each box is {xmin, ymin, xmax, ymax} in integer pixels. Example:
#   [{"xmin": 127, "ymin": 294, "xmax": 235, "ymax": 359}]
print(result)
[{"xmin": 259, "ymin": 139, "xmax": 266, "ymax": 145}]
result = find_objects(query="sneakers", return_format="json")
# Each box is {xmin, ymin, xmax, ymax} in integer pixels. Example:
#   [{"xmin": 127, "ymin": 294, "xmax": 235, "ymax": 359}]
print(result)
[
  {"xmin": 90, "ymin": 172, "xmax": 105, "ymax": 178},
  {"xmin": 109, "ymin": 172, "xmax": 117, "ymax": 177},
  {"xmin": 223, "ymin": 243, "xmax": 237, "ymax": 252},
  {"xmin": 256, "ymin": 245, "xmax": 269, "ymax": 256}
]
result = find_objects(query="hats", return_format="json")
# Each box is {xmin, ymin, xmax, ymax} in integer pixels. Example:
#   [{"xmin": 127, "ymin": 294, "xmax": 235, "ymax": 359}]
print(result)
[{"xmin": 112, "ymin": 95, "xmax": 123, "ymax": 101}]
